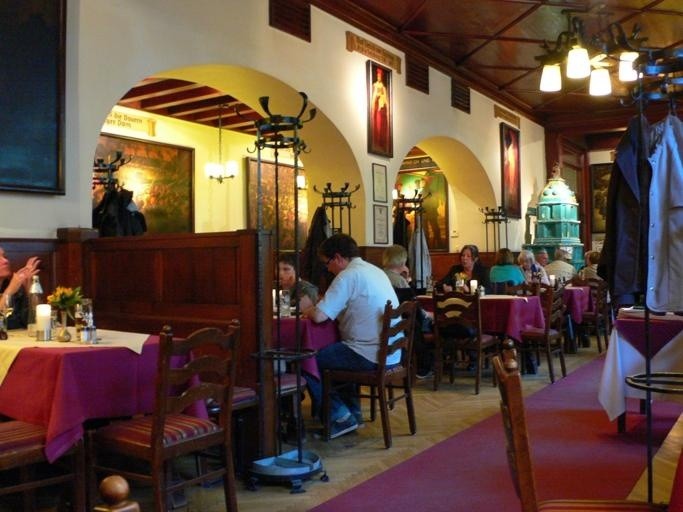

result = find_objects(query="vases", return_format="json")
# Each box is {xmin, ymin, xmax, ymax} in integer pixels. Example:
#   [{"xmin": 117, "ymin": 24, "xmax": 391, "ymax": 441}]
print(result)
[{"xmin": 56, "ymin": 313, "xmax": 75, "ymax": 344}]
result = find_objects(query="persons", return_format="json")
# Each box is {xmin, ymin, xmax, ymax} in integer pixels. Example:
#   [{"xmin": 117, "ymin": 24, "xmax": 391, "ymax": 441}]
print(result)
[
  {"xmin": 293, "ymin": 232, "xmax": 406, "ymax": 440},
  {"xmin": 0, "ymin": 246, "xmax": 42, "ymax": 332},
  {"xmin": 489, "ymin": 246, "xmax": 609, "ymax": 376},
  {"xmin": 370, "ymin": 67, "xmax": 393, "ymax": 154},
  {"xmin": 391, "ymin": 175, "xmax": 445, "ymax": 248},
  {"xmin": 433, "ymin": 244, "xmax": 490, "ymax": 372},
  {"xmin": 274, "ymin": 253, "xmax": 319, "ymax": 307},
  {"xmin": 381, "ymin": 243, "xmax": 434, "ymax": 379}
]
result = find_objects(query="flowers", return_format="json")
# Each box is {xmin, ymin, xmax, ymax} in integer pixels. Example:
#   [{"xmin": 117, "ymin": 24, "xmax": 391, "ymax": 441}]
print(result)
[{"xmin": 46, "ymin": 285, "xmax": 89, "ymax": 309}]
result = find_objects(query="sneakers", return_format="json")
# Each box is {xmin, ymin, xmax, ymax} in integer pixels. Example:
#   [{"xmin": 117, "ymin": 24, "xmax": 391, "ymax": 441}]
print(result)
[
  {"xmin": 416, "ymin": 368, "xmax": 432, "ymax": 379},
  {"xmin": 315, "ymin": 412, "xmax": 364, "ymax": 440}
]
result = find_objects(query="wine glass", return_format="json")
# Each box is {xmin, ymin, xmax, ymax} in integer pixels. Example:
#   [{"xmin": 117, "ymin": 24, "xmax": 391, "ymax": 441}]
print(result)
[{"xmin": 0, "ymin": 293, "xmax": 14, "ymax": 341}]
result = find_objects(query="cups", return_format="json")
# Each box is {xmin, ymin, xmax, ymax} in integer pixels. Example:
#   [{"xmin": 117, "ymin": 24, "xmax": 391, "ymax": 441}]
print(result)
[
  {"xmin": 74, "ymin": 298, "xmax": 94, "ymax": 342},
  {"xmin": 469, "ymin": 280, "xmax": 478, "ymax": 294},
  {"xmin": 456, "ymin": 280, "xmax": 464, "ymax": 293},
  {"xmin": 33, "ymin": 303, "xmax": 52, "ymax": 343},
  {"xmin": 278, "ymin": 289, "xmax": 291, "ymax": 317}
]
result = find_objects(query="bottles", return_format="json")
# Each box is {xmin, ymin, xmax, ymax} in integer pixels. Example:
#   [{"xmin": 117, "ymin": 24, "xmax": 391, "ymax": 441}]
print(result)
[
  {"xmin": 526, "ymin": 274, "xmax": 568, "ymax": 292},
  {"xmin": 26, "ymin": 275, "xmax": 43, "ymax": 337},
  {"xmin": 426, "ymin": 271, "xmax": 433, "ymax": 297},
  {"xmin": 83, "ymin": 327, "xmax": 95, "ymax": 344},
  {"xmin": 48, "ymin": 309, "xmax": 61, "ymax": 339},
  {"xmin": 479, "ymin": 286, "xmax": 486, "ymax": 298}
]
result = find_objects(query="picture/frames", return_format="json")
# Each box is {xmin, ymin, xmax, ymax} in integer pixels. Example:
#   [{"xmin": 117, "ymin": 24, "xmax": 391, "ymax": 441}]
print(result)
[
  {"xmin": 372, "ymin": 163, "xmax": 387, "ymax": 203},
  {"xmin": 500, "ymin": 122, "xmax": 521, "ymax": 218},
  {"xmin": 0, "ymin": 0, "xmax": 66, "ymax": 195},
  {"xmin": 366, "ymin": 60, "xmax": 393, "ymax": 157},
  {"xmin": 373, "ymin": 204, "xmax": 388, "ymax": 244}
]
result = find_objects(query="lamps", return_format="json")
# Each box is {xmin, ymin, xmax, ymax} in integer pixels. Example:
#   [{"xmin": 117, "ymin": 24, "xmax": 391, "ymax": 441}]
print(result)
[
  {"xmin": 205, "ymin": 102, "xmax": 238, "ymax": 183},
  {"xmin": 534, "ymin": 1, "xmax": 648, "ymax": 99}
]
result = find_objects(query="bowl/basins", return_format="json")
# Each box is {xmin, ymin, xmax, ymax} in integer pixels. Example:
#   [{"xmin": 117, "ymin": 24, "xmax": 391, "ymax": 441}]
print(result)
[{"xmin": 56, "ymin": 227, "xmax": 97, "ymax": 242}]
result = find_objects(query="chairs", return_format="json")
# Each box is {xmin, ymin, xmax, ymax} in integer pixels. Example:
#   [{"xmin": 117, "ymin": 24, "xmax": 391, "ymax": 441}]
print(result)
[
  {"xmin": 490, "ymin": 349, "xmax": 666, "ymax": 512},
  {"xmin": 1, "ymin": 418, "xmax": 90, "ymax": 512},
  {"xmin": 94, "ymin": 316, "xmax": 241, "ymax": 512},
  {"xmin": 274, "ymin": 280, "xmax": 609, "ymax": 449}
]
